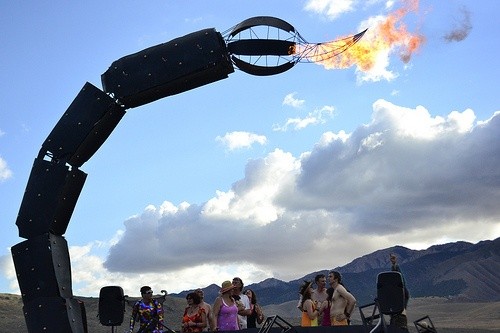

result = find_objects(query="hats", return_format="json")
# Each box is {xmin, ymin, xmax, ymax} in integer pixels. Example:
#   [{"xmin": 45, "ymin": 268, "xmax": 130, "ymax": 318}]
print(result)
[{"xmin": 220, "ymin": 281, "xmax": 236, "ymax": 293}]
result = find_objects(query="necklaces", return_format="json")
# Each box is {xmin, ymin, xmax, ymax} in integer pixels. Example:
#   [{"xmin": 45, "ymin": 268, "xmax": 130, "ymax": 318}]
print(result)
[{"xmin": 188, "ymin": 307, "xmax": 196, "ymax": 316}]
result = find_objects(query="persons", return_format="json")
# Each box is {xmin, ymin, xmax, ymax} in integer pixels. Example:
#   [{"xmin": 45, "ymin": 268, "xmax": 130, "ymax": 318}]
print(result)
[
  {"xmin": 231, "ymin": 277, "xmax": 252, "ymax": 329},
  {"xmin": 311, "ymin": 274, "xmax": 328, "ymax": 317},
  {"xmin": 243, "ymin": 289, "xmax": 264, "ymax": 328},
  {"xmin": 194, "ymin": 288, "xmax": 213, "ymax": 331},
  {"xmin": 328, "ymin": 271, "xmax": 356, "ymax": 325},
  {"xmin": 128, "ymin": 286, "xmax": 164, "ymax": 333},
  {"xmin": 299, "ymin": 280, "xmax": 321, "ymax": 327},
  {"xmin": 210, "ymin": 281, "xmax": 241, "ymax": 331},
  {"xmin": 181, "ymin": 292, "xmax": 207, "ymax": 332},
  {"xmin": 319, "ymin": 287, "xmax": 332, "ymax": 326}
]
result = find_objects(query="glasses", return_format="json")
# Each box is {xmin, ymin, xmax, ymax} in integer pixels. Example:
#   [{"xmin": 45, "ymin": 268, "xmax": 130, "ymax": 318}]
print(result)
[
  {"xmin": 145, "ymin": 291, "xmax": 153, "ymax": 294},
  {"xmin": 186, "ymin": 298, "xmax": 191, "ymax": 300}
]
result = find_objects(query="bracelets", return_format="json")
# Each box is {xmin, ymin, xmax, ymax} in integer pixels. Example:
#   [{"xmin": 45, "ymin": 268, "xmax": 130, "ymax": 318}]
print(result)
[{"xmin": 315, "ymin": 306, "xmax": 320, "ymax": 311}]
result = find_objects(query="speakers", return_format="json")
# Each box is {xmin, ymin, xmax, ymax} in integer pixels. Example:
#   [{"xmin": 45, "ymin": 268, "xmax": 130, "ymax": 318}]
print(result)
[{"xmin": 98, "ymin": 285, "xmax": 124, "ymax": 325}]
